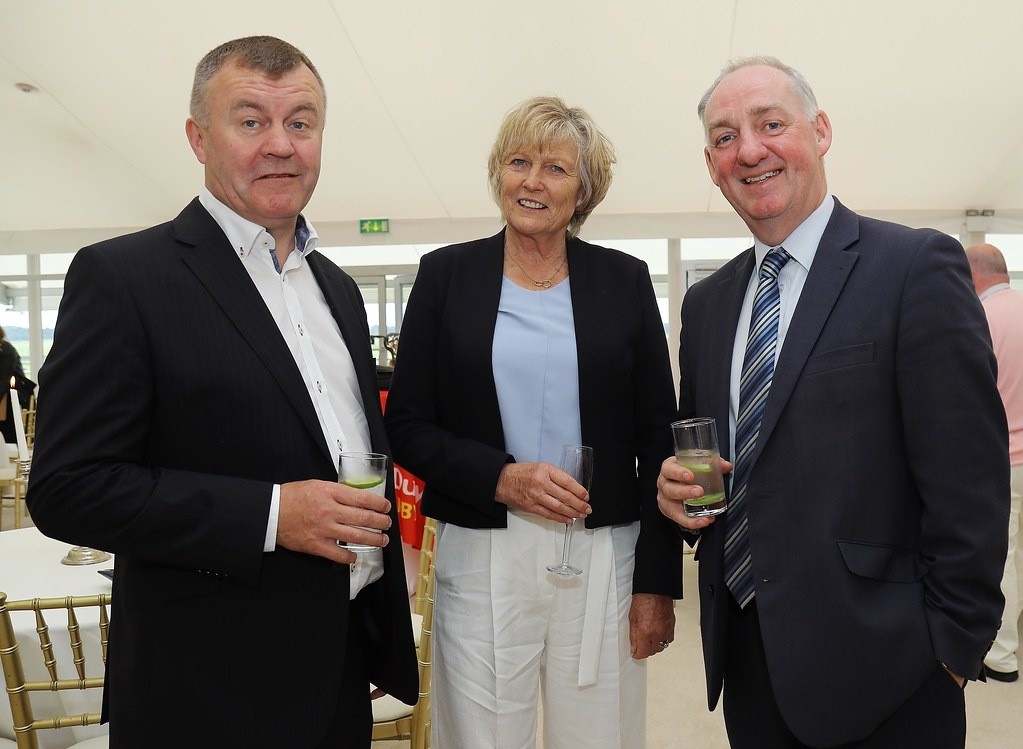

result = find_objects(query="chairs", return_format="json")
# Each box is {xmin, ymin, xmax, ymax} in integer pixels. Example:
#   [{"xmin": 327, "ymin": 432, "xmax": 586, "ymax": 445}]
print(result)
[{"xmin": 0, "ymin": 398, "xmax": 439, "ymax": 749}]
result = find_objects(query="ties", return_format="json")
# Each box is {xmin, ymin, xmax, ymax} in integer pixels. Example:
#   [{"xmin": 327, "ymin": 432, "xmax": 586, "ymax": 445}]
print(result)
[{"xmin": 724, "ymin": 246, "xmax": 793, "ymax": 610}]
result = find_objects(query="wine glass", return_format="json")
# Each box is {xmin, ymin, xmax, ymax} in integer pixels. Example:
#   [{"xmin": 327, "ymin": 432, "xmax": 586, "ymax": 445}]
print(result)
[{"xmin": 545, "ymin": 444, "xmax": 593, "ymax": 576}]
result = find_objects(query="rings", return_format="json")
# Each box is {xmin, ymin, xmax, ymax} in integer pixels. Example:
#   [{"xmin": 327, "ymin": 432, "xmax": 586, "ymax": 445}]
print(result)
[{"xmin": 660, "ymin": 641, "xmax": 669, "ymax": 649}]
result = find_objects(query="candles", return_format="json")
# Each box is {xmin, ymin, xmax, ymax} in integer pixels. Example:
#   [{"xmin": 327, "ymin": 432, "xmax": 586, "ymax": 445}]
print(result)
[{"xmin": 10, "ymin": 377, "xmax": 29, "ymax": 458}]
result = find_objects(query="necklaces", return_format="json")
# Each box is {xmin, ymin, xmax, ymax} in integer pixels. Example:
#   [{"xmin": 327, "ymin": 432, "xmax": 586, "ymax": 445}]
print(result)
[{"xmin": 502, "ymin": 242, "xmax": 568, "ymax": 290}]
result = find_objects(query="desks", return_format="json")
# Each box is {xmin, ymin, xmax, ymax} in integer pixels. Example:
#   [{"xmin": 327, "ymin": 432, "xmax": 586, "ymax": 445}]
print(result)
[{"xmin": 0, "ymin": 528, "xmax": 116, "ymax": 749}]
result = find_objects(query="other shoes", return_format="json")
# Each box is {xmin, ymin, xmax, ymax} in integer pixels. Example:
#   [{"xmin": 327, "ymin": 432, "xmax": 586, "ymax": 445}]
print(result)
[{"xmin": 982, "ymin": 664, "xmax": 1018, "ymax": 682}]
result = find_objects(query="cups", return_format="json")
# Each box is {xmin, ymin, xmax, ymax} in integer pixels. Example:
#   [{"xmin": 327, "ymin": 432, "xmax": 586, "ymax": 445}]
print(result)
[
  {"xmin": 671, "ymin": 416, "xmax": 729, "ymax": 519},
  {"xmin": 335, "ymin": 451, "xmax": 389, "ymax": 553}
]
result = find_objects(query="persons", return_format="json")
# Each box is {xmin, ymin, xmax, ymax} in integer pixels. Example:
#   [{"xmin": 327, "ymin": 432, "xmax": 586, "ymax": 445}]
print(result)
[
  {"xmin": 657, "ymin": 54, "xmax": 1011, "ymax": 749},
  {"xmin": 27, "ymin": 35, "xmax": 421, "ymax": 749},
  {"xmin": 967, "ymin": 244, "xmax": 1023, "ymax": 685},
  {"xmin": 0, "ymin": 329, "xmax": 37, "ymax": 443},
  {"xmin": 384, "ymin": 96, "xmax": 685, "ymax": 749}
]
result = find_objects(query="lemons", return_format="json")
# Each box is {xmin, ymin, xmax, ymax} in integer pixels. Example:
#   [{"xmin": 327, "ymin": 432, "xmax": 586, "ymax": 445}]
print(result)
[
  {"xmin": 685, "ymin": 493, "xmax": 723, "ymax": 504},
  {"xmin": 680, "ymin": 464, "xmax": 713, "ymax": 473},
  {"xmin": 341, "ymin": 476, "xmax": 384, "ymax": 490}
]
